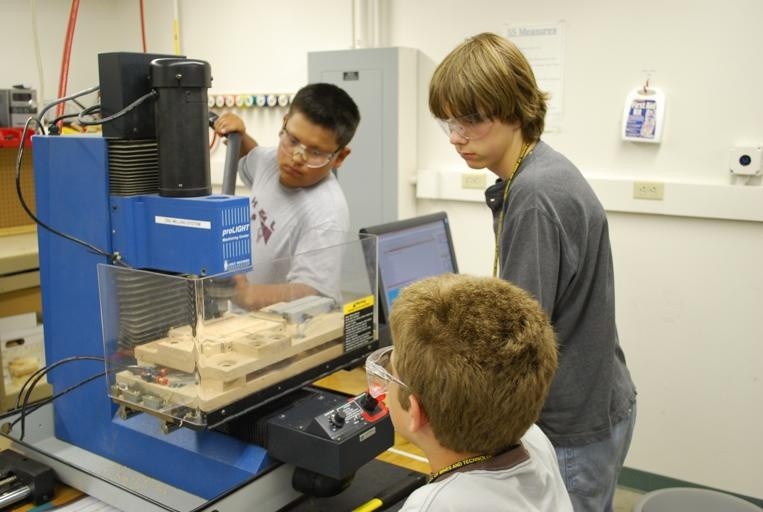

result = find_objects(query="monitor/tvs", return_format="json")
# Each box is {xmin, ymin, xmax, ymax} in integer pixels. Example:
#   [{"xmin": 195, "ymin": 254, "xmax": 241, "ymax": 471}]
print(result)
[{"xmin": 359, "ymin": 211, "xmax": 459, "ymax": 343}]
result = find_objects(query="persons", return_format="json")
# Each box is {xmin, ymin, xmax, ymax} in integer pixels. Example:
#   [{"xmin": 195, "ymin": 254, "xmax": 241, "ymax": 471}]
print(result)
[
  {"xmin": 210, "ymin": 81, "xmax": 362, "ymax": 310},
  {"xmin": 426, "ymin": 31, "xmax": 639, "ymax": 511},
  {"xmin": 360, "ymin": 271, "xmax": 575, "ymax": 511}
]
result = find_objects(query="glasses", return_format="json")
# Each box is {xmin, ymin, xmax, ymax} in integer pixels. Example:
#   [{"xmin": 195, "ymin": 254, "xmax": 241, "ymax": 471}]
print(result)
[
  {"xmin": 438, "ymin": 109, "xmax": 501, "ymax": 142},
  {"xmin": 364, "ymin": 346, "xmax": 432, "ymax": 427},
  {"xmin": 278, "ymin": 116, "xmax": 345, "ymax": 169}
]
